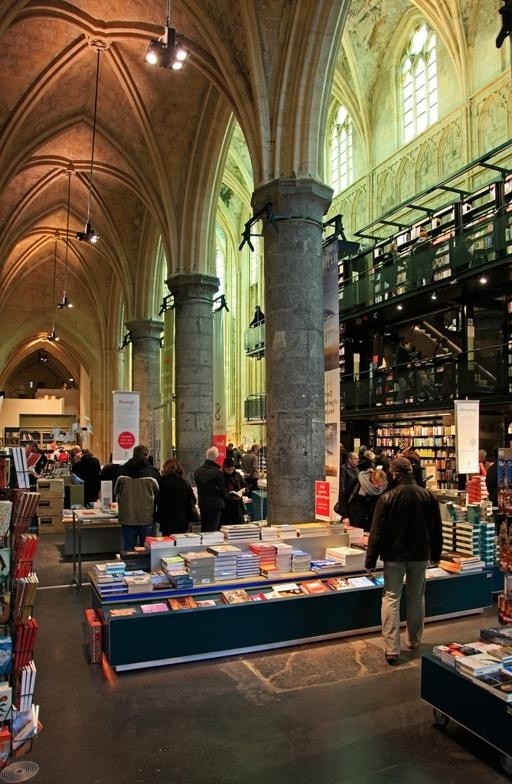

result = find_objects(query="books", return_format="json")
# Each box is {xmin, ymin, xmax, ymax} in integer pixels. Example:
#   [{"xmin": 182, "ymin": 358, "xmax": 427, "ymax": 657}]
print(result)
[
  {"xmin": 89, "ymin": 524, "xmax": 360, "ymax": 617},
  {"xmin": 437, "ymin": 489, "xmax": 486, "ymax": 576},
  {"xmin": 432, "ymin": 622, "xmax": 511, "ymax": 706},
  {"xmin": 1, "ymin": 427, "xmax": 67, "ymax": 759},
  {"xmin": 377, "ymin": 425, "xmax": 459, "ymax": 490}
]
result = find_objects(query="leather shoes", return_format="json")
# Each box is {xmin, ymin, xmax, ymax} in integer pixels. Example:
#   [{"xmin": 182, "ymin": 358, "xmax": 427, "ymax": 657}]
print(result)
[{"xmin": 385, "ymin": 654, "xmax": 398, "ymax": 660}]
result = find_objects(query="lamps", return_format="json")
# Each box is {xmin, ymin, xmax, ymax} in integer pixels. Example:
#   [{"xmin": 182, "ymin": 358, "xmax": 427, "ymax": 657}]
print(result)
[
  {"xmin": 144, "ymin": 1, "xmax": 190, "ymax": 73},
  {"xmin": 39, "ymin": 48, "xmax": 103, "ymax": 361}
]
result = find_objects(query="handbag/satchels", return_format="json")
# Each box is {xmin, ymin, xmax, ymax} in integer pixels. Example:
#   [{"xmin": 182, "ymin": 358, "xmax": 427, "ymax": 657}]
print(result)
[
  {"xmin": 333, "ymin": 483, "xmax": 360, "ymax": 517},
  {"xmin": 184, "ymin": 479, "xmax": 200, "ymax": 522}
]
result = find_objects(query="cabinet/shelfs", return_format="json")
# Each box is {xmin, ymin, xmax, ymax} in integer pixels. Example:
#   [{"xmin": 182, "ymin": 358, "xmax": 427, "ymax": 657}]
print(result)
[
  {"xmin": 339, "ymin": 170, "xmax": 512, "ymax": 305},
  {"xmin": 366, "ymin": 400, "xmax": 502, "ymax": 489},
  {"xmin": 0, "ymin": 425, "xmax": 81, "ymax": 783}
]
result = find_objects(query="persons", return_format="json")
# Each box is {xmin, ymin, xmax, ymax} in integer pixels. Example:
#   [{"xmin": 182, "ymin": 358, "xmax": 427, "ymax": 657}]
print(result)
[
  {"xmin": 68, "ymin": 443, "xmax": 267, "ymax": 551},
  {"xmin": 374, "ymin": 243, "xmax": 406, "ymax": 298},
  {"xmin": 454, "ymin": 237, "xmax": 475, "ymax": 273},
  {"xmin": 339, "ymin": 441, "xmax": 425, "ymax": 530},
  {"xmin": 364, "ymin": 453, "xmax": 443, "ymax": 664},
  {"xmin": 393, "ymin": 335, "xmax": 413, "ymax": 406},
  {"xmin": 478, "ymin": 449, "xmax": 498, "ymax": 505},
  {"xmin": 249, "ymin": 305, "xmax": 265, "ymax": 329},
  {"xmin": 407, "ymin": 231, "xmax": 437, "ymax": 288}
]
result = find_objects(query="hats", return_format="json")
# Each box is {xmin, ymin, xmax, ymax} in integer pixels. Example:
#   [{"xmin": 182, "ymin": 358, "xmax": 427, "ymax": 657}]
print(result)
[
  {"xmin": 222, "ymin": 457, "xmax": 235, "ymax": 468},
  {"xmin": 389, "ymin": 456, "xmax": 412, "ymax": 474}
]
